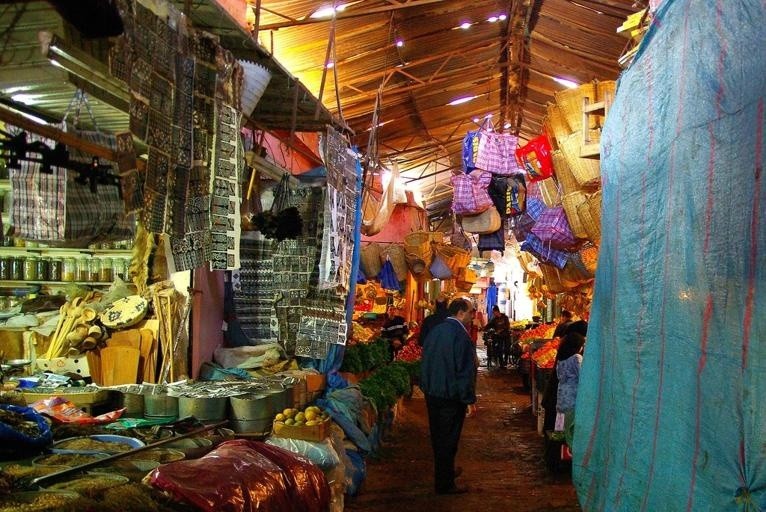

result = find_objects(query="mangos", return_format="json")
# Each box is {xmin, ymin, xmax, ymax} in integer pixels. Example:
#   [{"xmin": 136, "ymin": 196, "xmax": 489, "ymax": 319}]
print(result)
[{"xmin": 352, "ymin": 321, "xmax": 374, "ymax": 345}]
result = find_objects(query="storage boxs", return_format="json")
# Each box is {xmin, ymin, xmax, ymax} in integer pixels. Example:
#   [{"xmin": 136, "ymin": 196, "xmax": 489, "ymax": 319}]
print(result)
[{"xmin": 269, "ymin": 416, "xmax": 333, "ymax": 443}]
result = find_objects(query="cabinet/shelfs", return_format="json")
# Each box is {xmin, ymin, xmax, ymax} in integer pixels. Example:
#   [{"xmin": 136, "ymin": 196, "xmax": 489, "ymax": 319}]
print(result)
[{"xmin": 0, "ymin": 246, "xmax": 141, "ymax": 359}]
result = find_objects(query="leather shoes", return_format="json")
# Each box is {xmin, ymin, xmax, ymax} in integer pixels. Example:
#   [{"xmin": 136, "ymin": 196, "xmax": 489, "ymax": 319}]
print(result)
[{"xmin": 434, "ymin": 465, "xmax": 469, "ymax": 495}]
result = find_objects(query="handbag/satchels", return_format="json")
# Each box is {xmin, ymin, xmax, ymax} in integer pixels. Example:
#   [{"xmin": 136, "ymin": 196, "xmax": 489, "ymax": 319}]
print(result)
[
  {"xmin": 9, "ymin": 88, "xmax": 123, "ymax": 245},
  {"xmin": 462, "ymin": 119, "xmax": 522, "ymax": 176},
  {"xmin": 452, "ymin": 170, "xmax": 527, "ymax": 251}
]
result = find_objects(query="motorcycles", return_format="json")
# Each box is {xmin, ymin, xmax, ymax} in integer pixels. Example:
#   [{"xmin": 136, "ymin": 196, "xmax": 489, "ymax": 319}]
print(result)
[{"xmin": 478, "ymin": 326, "xmax": 502, "ymax": 368}]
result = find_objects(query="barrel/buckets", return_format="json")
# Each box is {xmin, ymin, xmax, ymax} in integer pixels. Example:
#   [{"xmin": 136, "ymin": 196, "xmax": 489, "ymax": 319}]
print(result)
[{"xmin": 123, "ymin": 371, "xmax": 324, "ymax": 434}]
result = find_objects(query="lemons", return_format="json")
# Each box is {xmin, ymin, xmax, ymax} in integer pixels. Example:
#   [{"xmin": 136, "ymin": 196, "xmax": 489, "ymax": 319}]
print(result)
[{"xmin": 535, "ymin": 349, "xmax": 557, "ymax": 366}]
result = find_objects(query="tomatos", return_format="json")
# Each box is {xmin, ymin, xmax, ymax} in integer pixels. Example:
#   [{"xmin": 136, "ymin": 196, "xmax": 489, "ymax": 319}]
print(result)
[{"xmin": 540, "ymin": 359, "xmax": 555, "ymax": 369}]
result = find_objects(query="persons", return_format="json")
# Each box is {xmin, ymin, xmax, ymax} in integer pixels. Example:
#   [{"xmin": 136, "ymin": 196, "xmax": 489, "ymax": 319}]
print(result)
[
  {"xmin": 379, "ymin": 303, "xmax": 410, "ymax": 365},
  {"xmin": 554, "ymin": 332, "xmax": 585, "ymax": 464},
  {"xmin": 552, "ymin": 309, "xmax": 574, "ymax": 338},
  {"xmin": 539, "ymin": 319, "xmax": 587, "ymax": 474},
  {"xmin": 418, "ymin": 297, "xmax": 479, "ymax": 494},
  {"xmin": 416, "ymin": 296, "xmax": 511, "ymax": 369}
]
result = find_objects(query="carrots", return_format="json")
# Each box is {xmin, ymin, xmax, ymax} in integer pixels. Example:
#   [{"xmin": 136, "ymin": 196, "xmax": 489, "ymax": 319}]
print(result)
[{"xmin": 532, "ymin": 339, "xmax": 559, "ymax": 358}]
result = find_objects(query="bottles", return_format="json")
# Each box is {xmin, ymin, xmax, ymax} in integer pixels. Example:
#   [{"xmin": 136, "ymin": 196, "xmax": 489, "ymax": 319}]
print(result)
[
  {"xmin": 0, "ymin": 230, "xmax": 132, "ymax": 284},
  {"xmin": 1, "ymin": 296, "xmax": 19, "ymax": 311}
]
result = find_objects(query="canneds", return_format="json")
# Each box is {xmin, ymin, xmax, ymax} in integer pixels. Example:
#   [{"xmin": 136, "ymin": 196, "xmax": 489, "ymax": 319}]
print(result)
[{"xmin": 1, "ymin": 256, "xmax": 135, "ymax": 282}]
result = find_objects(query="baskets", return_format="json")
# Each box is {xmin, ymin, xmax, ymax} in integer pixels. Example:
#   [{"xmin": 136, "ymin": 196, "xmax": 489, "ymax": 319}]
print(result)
[
  {"xmin": 233, "ymin": 57, "xmax": 272, "ymax": 130},
  {"xmin": 359, "ymin": 231, "xmax": 477, "ymax": 292},
  {"xmin": 275, "ymin": 368, "xmax": 322, "ymax": 408},
  {"xmin": 516, "ymin": 76, "xmax": 617, "ymax": 295},
  {"xmin": 270, "ymin": 405, "xmax": 333, "ymax": 444}
]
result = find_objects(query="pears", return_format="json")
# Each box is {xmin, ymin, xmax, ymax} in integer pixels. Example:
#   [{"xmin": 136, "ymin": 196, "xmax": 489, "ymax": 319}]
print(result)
[{"xmin": 276, "ymin": 405, "xmax": 327, "ymax": 426}]
